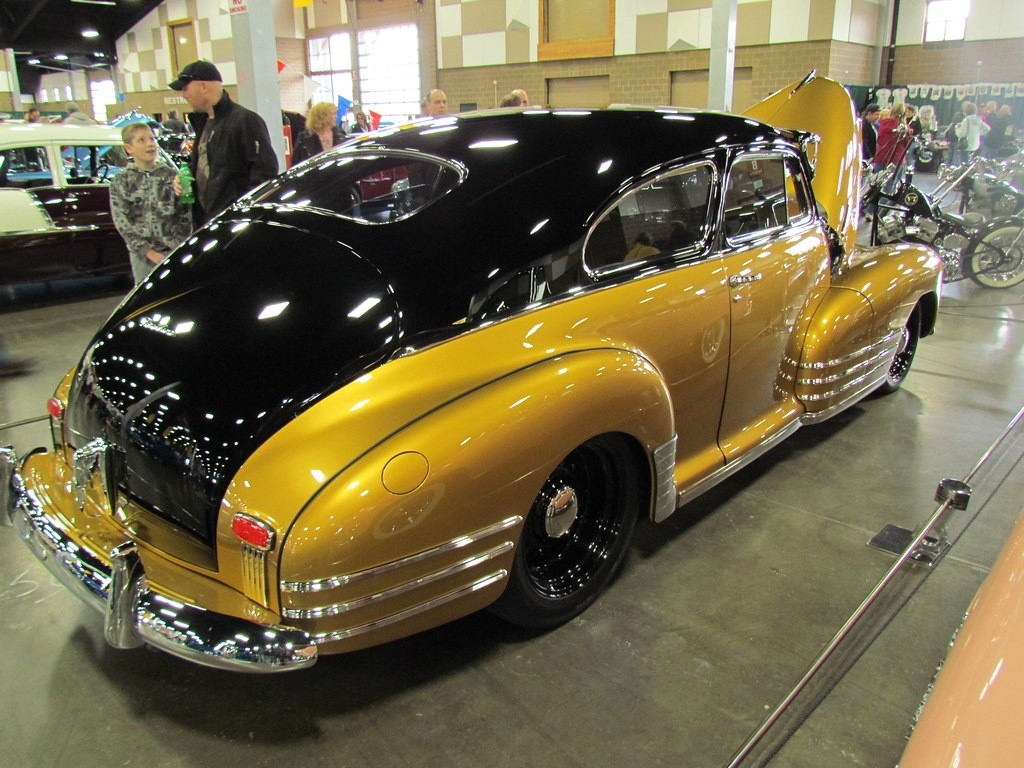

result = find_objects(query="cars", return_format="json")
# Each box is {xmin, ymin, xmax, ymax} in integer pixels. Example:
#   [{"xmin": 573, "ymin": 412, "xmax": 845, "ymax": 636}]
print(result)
[
  {"xmin": 0, "ymin": 124, "xmax": 183, "ymax": 288},
  {"xmin": 5, "ymin": 108, "xmax": 949, "ymax": 672},
  {"xmin": 155, "ymin": 120, "xmax": 195, "ymax": 164}
]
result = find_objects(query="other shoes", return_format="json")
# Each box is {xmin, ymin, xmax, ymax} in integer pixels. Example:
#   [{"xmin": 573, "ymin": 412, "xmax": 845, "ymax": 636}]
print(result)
[{"xmin": 865, "ymin": 213, "xmax": 873, "ymax": 222}]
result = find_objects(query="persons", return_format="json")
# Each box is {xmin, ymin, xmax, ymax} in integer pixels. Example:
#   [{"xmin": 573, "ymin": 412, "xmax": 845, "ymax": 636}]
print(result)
[
  {"xmin": 624, "ymin": 230, "xmax": 661, "ymax": 261},
  {"xmin": 659, "ymin": 219, "xmax": 700, "ymax": 252},
  {"xmin": 167, "ymin": 61, "xmax": 279, "ymax": 232},
  {"xmin": 419, "ymin": 88, "xmax": 448, "ymax": 117},
  {"xmin": 861, "ymin": 102, "xmax": 913, "ymax": 222},
  {"xmin": 351, "ymin": 111, "xmax": 372, "ymax": 133},
  {"xmin": 62, "ymin": 102, "xmax": 97, "ymax": 126},
  {"xmin": 902, "ymin": 106, "xmax": 922, "ymax": 172},
  {"xmin": 109, "ymin": 124, "xmax": 196, "ymax": 286},
  {"xmin": 290, "ymin": 98, "xmax": 349, "ymax": 166},
  {"xmin": 944, "ymin": 99, "xmax": 1013, "ymax": 165},
  {"xmin": 163, "ymin": 109, "xmax": 187, "ymax": 133},
  {"xmin": 499, "ymin": 89, "xmax": 529, "ymax": 108},
  {"xmin": 862, "ymin": 103, "xmax": 881, "ymax": 174},
  {"xmin": 879, "ymin": 108, "xmax": 891, "ymax": 120},
  {"xmin": 24, "ymin": 107, "xmax": 42, "ymax": 123}
]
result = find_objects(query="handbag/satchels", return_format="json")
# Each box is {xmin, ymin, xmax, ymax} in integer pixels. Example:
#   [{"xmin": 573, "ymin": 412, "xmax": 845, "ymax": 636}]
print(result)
[{"xmin": 955, "ymin": 117, "xmax": 969, "ymax": 150}]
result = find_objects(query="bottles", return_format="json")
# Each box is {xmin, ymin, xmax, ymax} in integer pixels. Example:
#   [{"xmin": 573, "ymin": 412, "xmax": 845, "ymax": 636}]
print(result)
[{"xmin": 178, "ymin": 162, "xmax": 195, "ymax": 204}]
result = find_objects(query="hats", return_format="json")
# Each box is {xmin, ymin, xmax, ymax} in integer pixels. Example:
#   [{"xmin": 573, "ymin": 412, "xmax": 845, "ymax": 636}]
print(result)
[
  {"xmin": 168, "ymin": 61, "xmax": 222, "ymax": 91},
  {"xmin": 866, "ymin": 104, "xmax": 882, "ymax": 111}
]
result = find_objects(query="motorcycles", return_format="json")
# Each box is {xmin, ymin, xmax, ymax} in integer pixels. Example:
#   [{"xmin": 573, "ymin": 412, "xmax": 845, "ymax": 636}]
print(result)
[{"xmin": 850, "ymin": 101, "xmax": 1024, "ymax": 290}]
